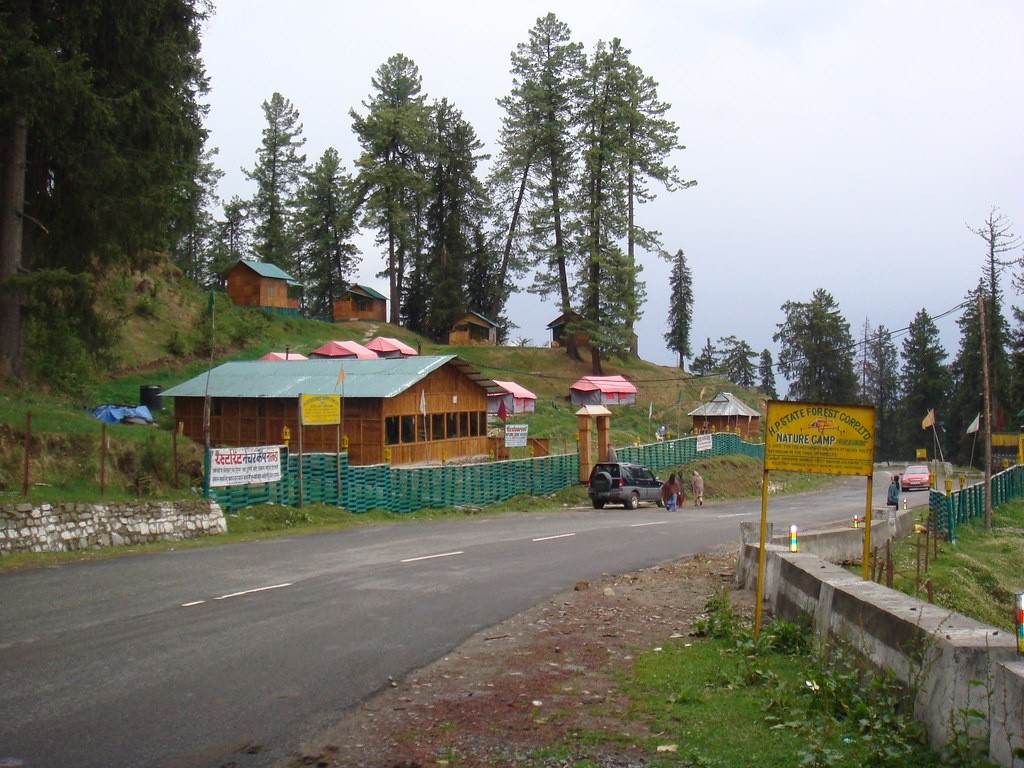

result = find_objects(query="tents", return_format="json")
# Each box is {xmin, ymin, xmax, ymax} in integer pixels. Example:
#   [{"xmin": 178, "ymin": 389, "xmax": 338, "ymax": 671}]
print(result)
[
  {"xmin": 258, "ymin": 336, "xmax": 418, "ymax": 361},
  {"xmin": 570, "ymin": 375, "xmax": 637, "ymax": 406},
  {"xmin": 487, "ymin": 380, "xmax": 537, "ymax": 416}
]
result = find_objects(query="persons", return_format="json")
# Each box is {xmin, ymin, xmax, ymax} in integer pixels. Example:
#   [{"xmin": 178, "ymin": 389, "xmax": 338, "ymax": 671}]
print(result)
[
  {"xmin": 886, "ymin": 476, "xmax": 899, "ymax": 511},
  {"xmin": 690, "ymin": 470, "xmax": 704, "ymax": 506},
  {"xmin": 661, "ymin": 472, "xmax": 685, "ymax": 511},
  {"xmin": 605, "ymin": 443, "xmax": 617, "ymax": 462}
]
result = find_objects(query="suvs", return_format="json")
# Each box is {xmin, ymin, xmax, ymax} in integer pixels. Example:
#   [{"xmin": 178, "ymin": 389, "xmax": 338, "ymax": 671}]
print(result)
[{"xmin": 588, "ymin": 462, "xmax": 666, "ymax": 510}]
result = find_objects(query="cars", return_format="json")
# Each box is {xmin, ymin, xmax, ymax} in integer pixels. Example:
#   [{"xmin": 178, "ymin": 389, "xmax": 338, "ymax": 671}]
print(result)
[{"xmin": 898, "ymin": 465, "xmax": 930, "ymax": 492}]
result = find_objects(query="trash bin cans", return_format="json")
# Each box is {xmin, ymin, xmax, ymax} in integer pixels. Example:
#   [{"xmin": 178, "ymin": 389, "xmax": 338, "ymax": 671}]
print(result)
[{"xmin": 140, "ymin": 383, "xmax": 163, "ymax": 416}]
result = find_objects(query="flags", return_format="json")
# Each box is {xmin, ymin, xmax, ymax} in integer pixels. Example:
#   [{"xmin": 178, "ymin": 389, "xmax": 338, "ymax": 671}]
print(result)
[
  {"xmin": 965, "ymin": 414, "xmax": 980, "ymax": 435},
  {"xmin": 922, "ymin": 409, "xmax": 935, "ymax": 430},
  {"xmin": 996, "ymin": 408, "xmax": 1004, "ymax": 432}
]
